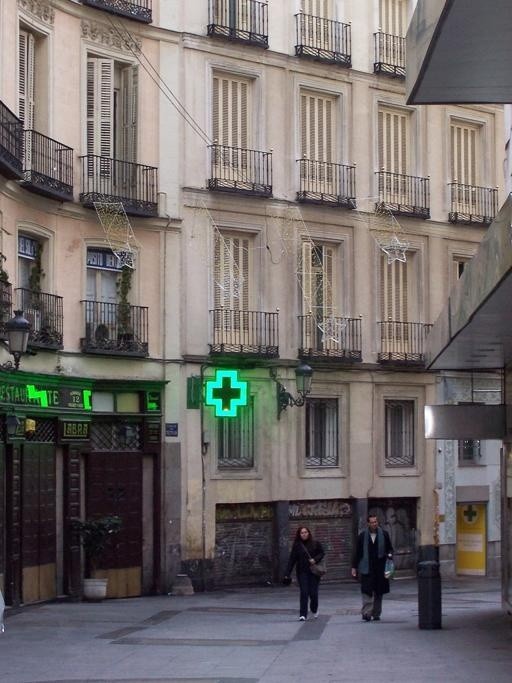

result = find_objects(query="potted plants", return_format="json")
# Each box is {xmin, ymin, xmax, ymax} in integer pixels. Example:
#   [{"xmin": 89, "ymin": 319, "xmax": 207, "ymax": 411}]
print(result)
[
  {"xmin": 71, "ymin": 515, "xmax": 122, "ymax": 604},
  {"xmin": 116, "ymin": 265, "xmax": 134, "ymax": 351}
]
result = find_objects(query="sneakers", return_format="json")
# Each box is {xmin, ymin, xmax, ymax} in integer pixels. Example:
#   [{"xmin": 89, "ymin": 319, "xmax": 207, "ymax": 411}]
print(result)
[
  {"xmin": 313, "ymin": 609, "xmax": 319, "ymax": 618},
  {"xmin": 363, "ymin": 615, "xmax": 379, "ymax": 621},
  {"xmin": 299, "ymin": 616, "xmax": 306, "ymax": 621}
]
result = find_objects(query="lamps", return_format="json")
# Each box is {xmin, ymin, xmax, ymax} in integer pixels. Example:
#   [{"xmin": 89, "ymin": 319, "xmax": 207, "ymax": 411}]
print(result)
[
  {"xmin": 0, "ymin": 309, "xmax": 31, "ymax": 373},
  {"xmin": 269, "ymin": 361, "xmax": 313, "ymax": 421},
  {"xmin": 5, "ymin": 406, "xmax": 20, "ymax": 437}
]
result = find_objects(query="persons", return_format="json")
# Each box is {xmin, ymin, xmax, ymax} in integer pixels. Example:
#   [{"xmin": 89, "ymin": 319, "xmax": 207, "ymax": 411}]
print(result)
[
  {"xmin": 349, "ymin": 515, "xmax": 394, "ymax": 621},
  {"xmin": 283, "ymin": 525, "xmax": 325, "ymax": 621}
]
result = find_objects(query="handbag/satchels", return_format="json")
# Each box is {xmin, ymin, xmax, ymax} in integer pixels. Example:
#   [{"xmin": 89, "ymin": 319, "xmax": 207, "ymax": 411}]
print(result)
[{"xmin": 309, "ymin": 560, "xmax": 327, "ymax": 576}]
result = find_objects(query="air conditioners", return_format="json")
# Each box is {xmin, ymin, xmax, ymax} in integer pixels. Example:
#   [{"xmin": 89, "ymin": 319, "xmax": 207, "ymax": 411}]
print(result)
[{"xmin": 86, "ymin": 322, "xmax": 115, "ymax": 349}]
